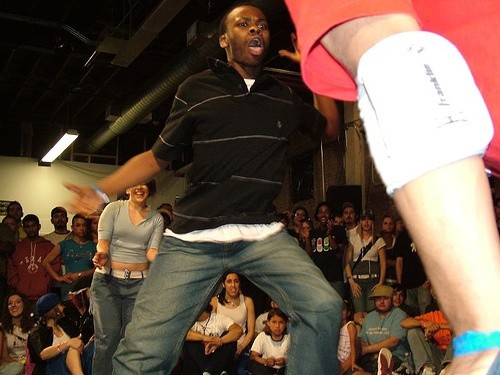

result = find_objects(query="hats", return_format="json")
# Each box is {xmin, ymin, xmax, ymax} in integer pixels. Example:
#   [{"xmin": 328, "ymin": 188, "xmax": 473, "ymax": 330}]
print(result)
[
  {"xmin": 360, "ymin": 210, "xmax": 375, "ymax": 219},
  {"xmin": 367, "ymin": 286, "xmax": 394, "ymax": 300},
  {"xmin": 36, "ymin": 292, "xmax": 61, "ymax": 314}
]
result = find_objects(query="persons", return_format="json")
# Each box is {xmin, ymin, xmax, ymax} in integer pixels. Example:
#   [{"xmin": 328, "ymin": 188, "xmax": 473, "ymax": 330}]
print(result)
[
  {"xmin": 62, "ymin": 5, "xmax": 344, "ymax": 375},
  {"xmin": 0, "ymin": 178, "xmax": 500, "ymax": 375},
  {"xmin": 285, "ymin": 1, "xmax": 500, "ymax": 375}
]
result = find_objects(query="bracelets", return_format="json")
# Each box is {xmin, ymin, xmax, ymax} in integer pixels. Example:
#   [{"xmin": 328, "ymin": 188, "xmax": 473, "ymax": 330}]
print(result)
[
  {"xmin": 451, "ymin": 331, "xmax": 500, "ymax": 357},
  {"xmin": 58, "ymin": 344, "xmax": 64, "ymax": 352},
  {"xmin": 79, "ymin": 272, "xmax": 84, "ymax": 278},
  {"xmin": 90, "ymin": 184, "xmax": 110, "ymax": 204}
]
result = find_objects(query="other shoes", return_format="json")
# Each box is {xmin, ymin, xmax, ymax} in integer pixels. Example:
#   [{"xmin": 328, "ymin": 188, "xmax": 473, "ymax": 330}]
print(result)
[{"xmin": 376, "ymin": 347, "xmax": 393, "ymax": 375}]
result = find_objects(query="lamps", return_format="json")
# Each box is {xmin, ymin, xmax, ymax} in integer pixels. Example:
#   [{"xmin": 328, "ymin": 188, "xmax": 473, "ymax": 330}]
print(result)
[{"xmin": 38, "ymin": 96, "xmax": 79, "ymax": 167}]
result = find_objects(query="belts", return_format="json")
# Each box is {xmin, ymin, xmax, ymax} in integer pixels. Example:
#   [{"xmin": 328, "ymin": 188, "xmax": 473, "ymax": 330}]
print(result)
[
  {"xmin": 352, "ymin": 274, "xmax": 378, "ymax": 279},
  {"xmin": 95, "ymin": 265, "xmax": 148, "ymax": 279}
]
